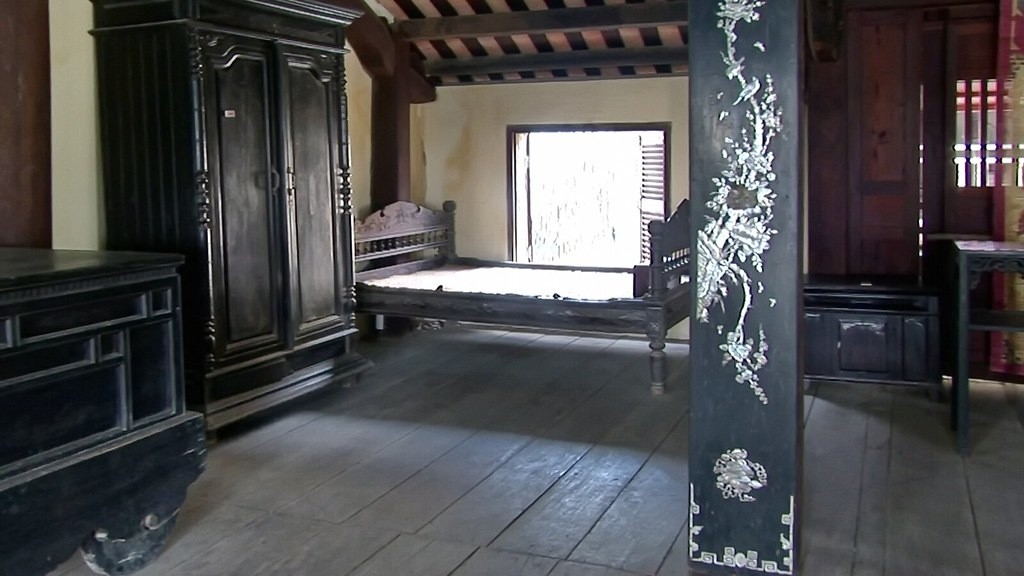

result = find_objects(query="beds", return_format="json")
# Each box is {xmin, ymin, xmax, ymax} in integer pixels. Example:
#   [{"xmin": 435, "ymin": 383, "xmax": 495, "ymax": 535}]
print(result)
[{"xmin": 353, "ymin": 198, "xmax": 693, "ymax": 396}]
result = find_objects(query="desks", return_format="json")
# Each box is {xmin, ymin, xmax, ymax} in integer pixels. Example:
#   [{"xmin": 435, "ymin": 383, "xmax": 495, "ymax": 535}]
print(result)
[
  {"xmin": 938, "ymin": 240, "xmax": 1024, "ymax": 457},
  {"xmin": 803, "ymin": 273, "xmax": 944, "ymax": 402},
  {"xmin": 0, "ymin": 247, "xmax": 207, "ymax": 576}
]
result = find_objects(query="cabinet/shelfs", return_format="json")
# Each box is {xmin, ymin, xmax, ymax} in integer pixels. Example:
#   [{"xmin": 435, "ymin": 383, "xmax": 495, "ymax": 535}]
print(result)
[{"xmin": 87, "ymin": 0, "xmax": 374, "ymax": 452}]
